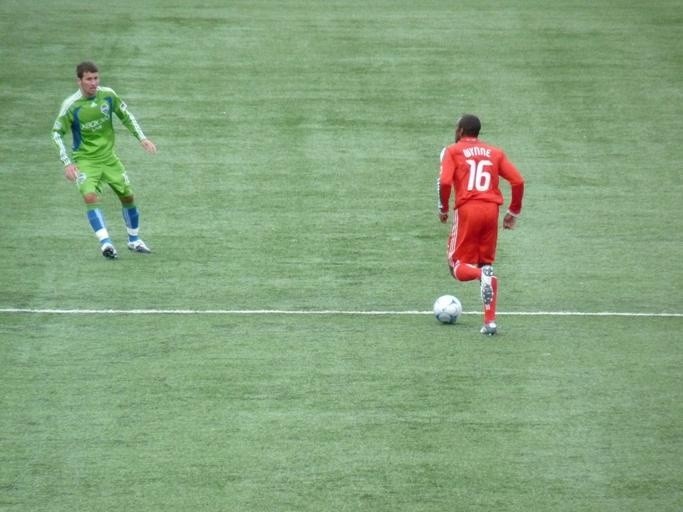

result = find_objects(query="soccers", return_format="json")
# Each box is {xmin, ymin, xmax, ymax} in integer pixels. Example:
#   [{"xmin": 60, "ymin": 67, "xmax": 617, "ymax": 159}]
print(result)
[{"xmin": 433, "ymin": 294, "xmax": 463, "ymax": 325}]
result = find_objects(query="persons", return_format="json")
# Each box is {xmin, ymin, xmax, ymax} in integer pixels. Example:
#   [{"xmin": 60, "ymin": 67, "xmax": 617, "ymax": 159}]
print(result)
[
  {"xmin": 435, "ymin": 113, "xmax": 525, "ymax": 337},
  {"xmin": 49, "ymin": 58, "xmax": 158, "ymax": 260}
]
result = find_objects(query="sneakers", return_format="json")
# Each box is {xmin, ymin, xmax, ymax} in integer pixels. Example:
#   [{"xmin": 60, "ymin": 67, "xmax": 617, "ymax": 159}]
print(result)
[
  {"xmin": 480, "ymin": 322, "xmax": 498, "ymax": 337},
  {"xmin": 126, "ymin": 238, "xmax": 152, "ymax": 254},
  {"xmin": 480, "ymin": 265, "xmax": 494, "ymax": 304},
  {"xmin": 100, "ymin": 243, "xmax": 118, "ymax": 260}
]
